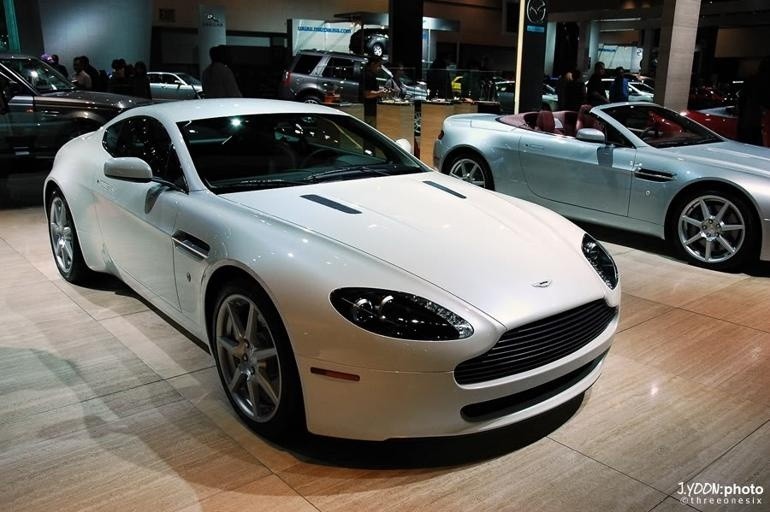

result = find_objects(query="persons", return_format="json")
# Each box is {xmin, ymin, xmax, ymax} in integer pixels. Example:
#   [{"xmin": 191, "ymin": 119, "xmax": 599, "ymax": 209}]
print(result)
[
  {"xmin": 555, "ymin": 72, "xmax": 572, "ymax": 111},
  {"xmin": 568, "ymin": 69, "xmax": 585, "ymax": 108},
  {"xmin": 382, "ymin": 72, "xmax": 407, "ymax": 99},
  {"xmin": 201, "ymin": 46, "xmax": 241, "ymax": 97},
  {"xmin": 41, "ymin": 53, "xmax": 152, "ymax": 100},
  {"xmin": 357, "ymin": 54, "xmax": 389, "ymax": 157},
  {"xmin": 735, "ymin": 55, "xmax": 770, "ymax": 146},
  {"xmin": 585, "ymin": 61, "xmax": 608, "ymax": 106},
  {"xmin": 609, "ymin": 66, "xmax": 629, "ymax": 101}
]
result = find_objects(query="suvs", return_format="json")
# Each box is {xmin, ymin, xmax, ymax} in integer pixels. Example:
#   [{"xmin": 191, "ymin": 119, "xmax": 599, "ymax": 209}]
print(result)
[
  {"xmin": 0, "ymin": 53, "xmax": 151, "ymax": 176},
  {"xmin": 350, "ymin": 28, "xmax": 390, "ymax": 57},
  {"xmin": 284, "ymin": 51, "xmax": 426, "ymax": 105}
]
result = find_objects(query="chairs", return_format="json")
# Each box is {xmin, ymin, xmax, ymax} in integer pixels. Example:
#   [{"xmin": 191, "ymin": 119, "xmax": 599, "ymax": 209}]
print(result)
[
  {"xmin": 270, "ymin": 141, "xmax": 315, "ymax": 170},
  {"xmin": 163, "ymin": 145, "xmax": 201, "ymax": 178},
  {"xmin": 533, "ymin": 103, "xmax": 606, "ymax": 139}
]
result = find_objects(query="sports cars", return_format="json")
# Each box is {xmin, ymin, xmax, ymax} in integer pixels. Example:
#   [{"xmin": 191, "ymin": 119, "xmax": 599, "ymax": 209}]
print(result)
[{"xmin": 432, "ymin": 103, "xmax": 769, "ymax": 267}]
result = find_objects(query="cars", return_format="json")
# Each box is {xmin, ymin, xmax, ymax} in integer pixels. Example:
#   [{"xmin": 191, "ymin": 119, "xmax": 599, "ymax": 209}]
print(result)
[
  {"xmin": 453, "ymin": 73, "xmax": 656, "ymax": 110},
  {"xmin": 45, "ymin": 98, "xmax": 622, "ymax": 446},
  {"xmin": 145, "ymin": 70, "xmax": 203, "ymax": 99}
]
